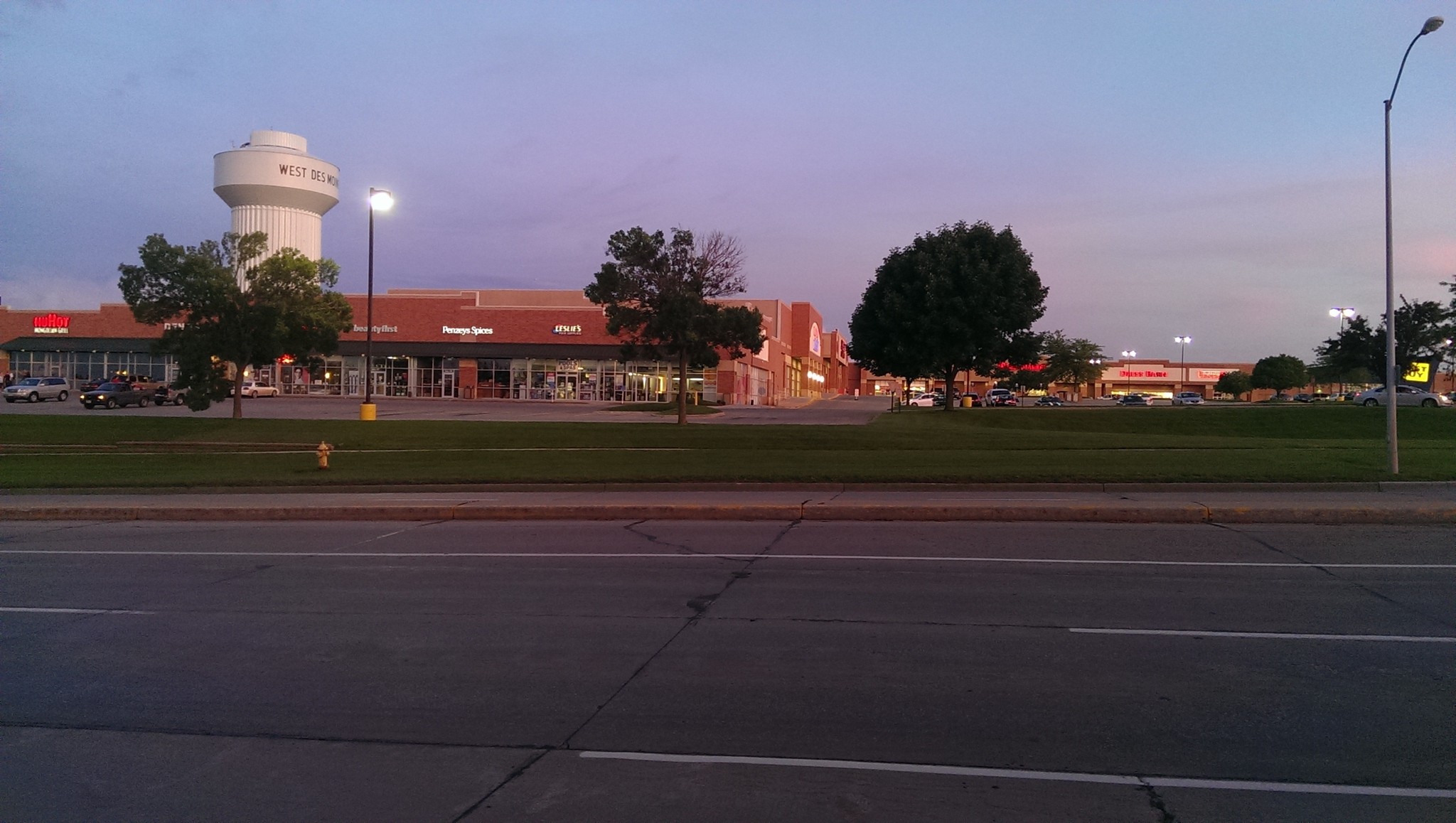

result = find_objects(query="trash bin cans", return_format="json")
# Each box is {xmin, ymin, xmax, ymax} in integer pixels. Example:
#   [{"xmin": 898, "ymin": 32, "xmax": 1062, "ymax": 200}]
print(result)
[{"xmin": 962, "ymin": 397, "xmax": 972, "ymax": 408}]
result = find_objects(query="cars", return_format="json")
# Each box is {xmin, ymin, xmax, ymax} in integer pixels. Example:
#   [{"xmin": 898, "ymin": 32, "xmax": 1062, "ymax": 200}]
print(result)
[
  {"xmin": 1034, "ymin": 396, "xmax": 1067, "ymax": 406},
  {"xmin": 900, "ymin": 389, "xmax": 947, "ymax": 407},
  {"xmin": 229, "ymin": 380, "xmax": 281, "ymax": 398},
  {"xmin": 992, "ymin": 394, "xmax": 1017, "ymax": 407},
  {"xmin": 1116, "ymin": 395, "xmax": 1147, "ymax": 406},
  {"xmin": 1171, "ymin": 391, "xmax": 1204, "ymax": 405},
  {"xmin": 1270, "ymin": 393, "xmax": 1294, "ymax": 402},
  {"xmin": 80, "ymin": 373, "xmax": 152, "ymax": 391},
  {"xmin": 1131, "ymin": 392, "xmax": 1152, "ymax": 406},
  {"xmin": 985, "ymin": 388, "xmax": 1015, "ymax": 407},
  {"xmin": 1353, "ymin": 384, "xmax": 1453, "ymax": 409},
  {"xmin": 1446, "ymin": 391, "xmax": 1456, "ymax": 402},
  {"xmin": 1099, "ymin": 394, "xmax": 1120, "ymax": 400},
  {"xmin": 1295, "ymin": 389, "xmax": 1365, "ymax": 403},
  {"xmin": 960, "ymin": 393, "xmax": 983, "ymax": 407},
  {"xmin": 952, "ymin": 388, "xmax": 961, "ymax": 400}
]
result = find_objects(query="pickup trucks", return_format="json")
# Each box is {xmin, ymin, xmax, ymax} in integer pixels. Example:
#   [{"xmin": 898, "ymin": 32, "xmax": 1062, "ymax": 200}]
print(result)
[
  {"xmin": 78, "ymin": 381, "xmax": 156, "ymax": 409},
  {"xmin": 148, "ymin": 381, "xmax": 195, "ymax": 406}
]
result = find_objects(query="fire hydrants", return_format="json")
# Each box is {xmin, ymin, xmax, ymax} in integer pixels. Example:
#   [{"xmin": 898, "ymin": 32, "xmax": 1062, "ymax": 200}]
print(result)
[{"xmin": 314, "ymin": 441, "xmax": 330, "ymax": 470}]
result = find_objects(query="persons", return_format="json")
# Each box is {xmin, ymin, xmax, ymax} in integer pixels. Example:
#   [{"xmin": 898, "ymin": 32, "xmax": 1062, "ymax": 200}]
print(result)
[
  {"xmin": 112, "ymin": 376, "xmax": 122, "ymax": 382},
  {"xmin": 293, "ymin": 367, "xmax": 304, "ymax": 388},
  {"xmin": 0, "ymin": 369, "xmax": 31, "ymax": 395}
]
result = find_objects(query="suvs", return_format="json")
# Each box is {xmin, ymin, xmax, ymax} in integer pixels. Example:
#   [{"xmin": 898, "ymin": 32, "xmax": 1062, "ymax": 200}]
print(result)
[{"xmin": 2, "ymin": 377, "xmax": 71, "ymax": 403}]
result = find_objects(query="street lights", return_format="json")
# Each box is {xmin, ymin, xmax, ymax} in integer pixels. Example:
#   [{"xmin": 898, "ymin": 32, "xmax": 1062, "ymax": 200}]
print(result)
[
  {"xmin": 1328, "ymin": 306, "xmax": 1355, "ymax": 401},
  {"xmin": 1378, "ymin": 12, "xmax": 1448, "ymax": 479},
  {"xmin": 1174, "ymin": 336, "xmax": 1192, "ymax": 392},
  {"xmin": 357, "ymin": 186, "xmax": 393, "ymax": 421},
  {"xmin": 1122, "ymin": 351, "xmax": 1138, "ymax": 397}
]
